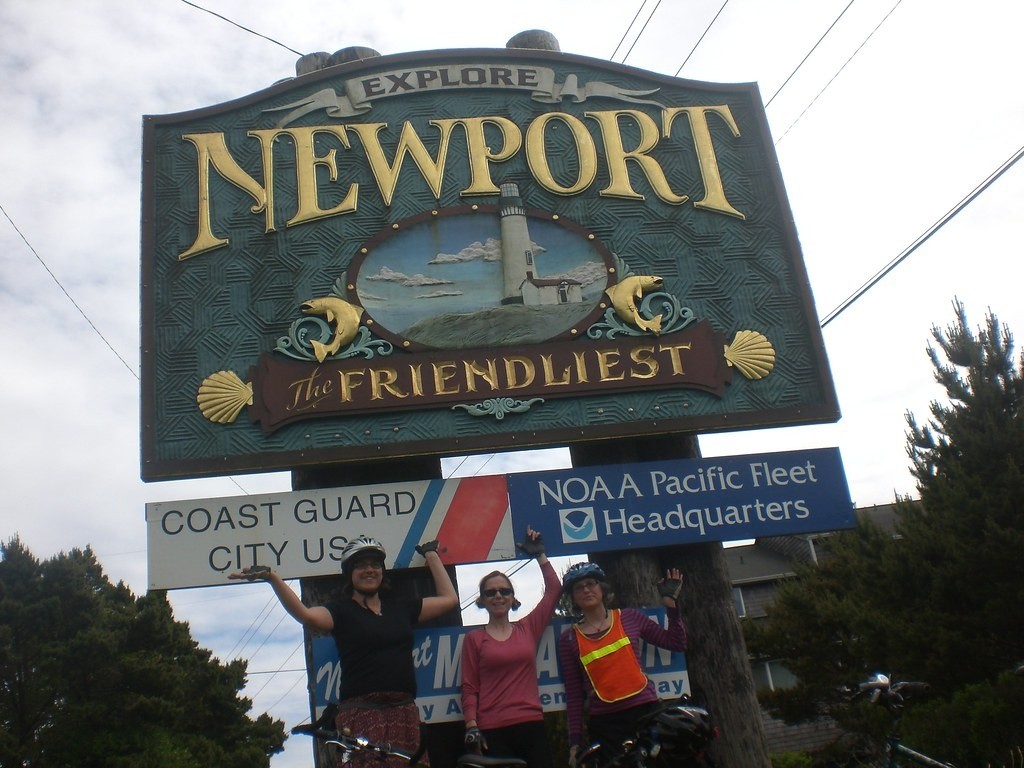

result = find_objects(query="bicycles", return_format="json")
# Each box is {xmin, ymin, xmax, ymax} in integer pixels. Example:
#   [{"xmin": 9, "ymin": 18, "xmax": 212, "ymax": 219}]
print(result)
[{"xmin": 290, "ymin": 693, "xmax": 719, "ymax": 768}]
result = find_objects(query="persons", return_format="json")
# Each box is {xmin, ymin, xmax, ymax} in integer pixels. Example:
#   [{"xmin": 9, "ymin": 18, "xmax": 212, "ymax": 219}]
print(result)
[
  {"xmin": 225, "ymin": 536, "xmax": 461, "ymax": 768},
  {"xmin": 460, "ymin": 523, "xmax": 565, "ymax": 768},
  {"xmin": 556, "ymin": 562, "xmax": 689, "ymax": 768}
]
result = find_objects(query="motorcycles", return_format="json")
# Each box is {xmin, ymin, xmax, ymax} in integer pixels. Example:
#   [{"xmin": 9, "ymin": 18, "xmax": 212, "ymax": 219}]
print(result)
[{"xmin": 824, "ymin": 654, "xmax": 931, "ymax": 768}]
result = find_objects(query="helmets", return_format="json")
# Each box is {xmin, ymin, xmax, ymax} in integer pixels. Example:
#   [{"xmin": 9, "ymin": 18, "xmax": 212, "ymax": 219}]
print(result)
[
  {"xmin": 563, "ymin": 562, "xmax": 605, "ymax": 589},
  {"xmin": 340, "ymin": 535, "xmax": 386, "ymax": 577}
]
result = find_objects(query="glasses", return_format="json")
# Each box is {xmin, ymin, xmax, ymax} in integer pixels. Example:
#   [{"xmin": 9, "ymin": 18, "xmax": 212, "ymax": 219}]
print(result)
[
  {"xmin": 352, "ymin": 558, "xmax": 383, "ymax": 570},
  {"xmin": 483, "ymin": 588, "xmax": 514, "ymax": 597},
  {"xmin": 569, "ymin": 579, "xmax": 600, "ymax": 593}
]
